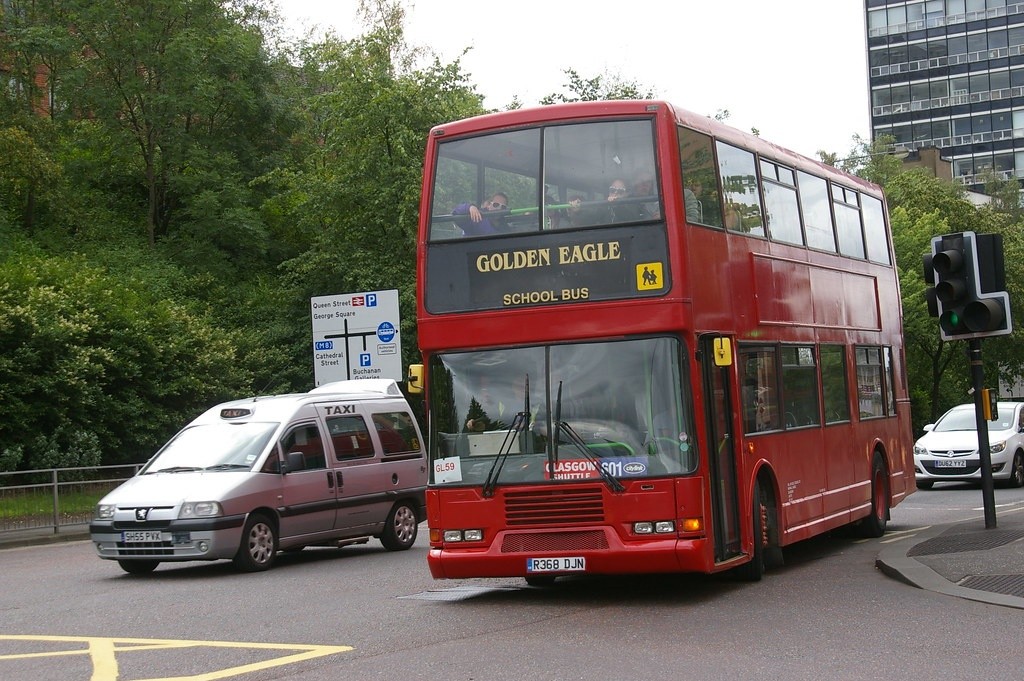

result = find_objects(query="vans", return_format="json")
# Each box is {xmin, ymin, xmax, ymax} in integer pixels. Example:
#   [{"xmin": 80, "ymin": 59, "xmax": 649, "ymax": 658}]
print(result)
[{"xmin": 90, "ymin": 378, "xmax": 428, "ymax": 572}]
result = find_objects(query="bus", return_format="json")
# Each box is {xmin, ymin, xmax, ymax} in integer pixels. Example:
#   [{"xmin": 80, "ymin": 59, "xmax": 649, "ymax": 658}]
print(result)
[{"xmin": 408, "ymin": 95, "xmax": 917, "ymax": 580}]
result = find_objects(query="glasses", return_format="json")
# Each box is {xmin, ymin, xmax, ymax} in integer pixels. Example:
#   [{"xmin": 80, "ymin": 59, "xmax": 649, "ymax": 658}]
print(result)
[
  {"xmin": 609, "ymin": 185, "xmax": 628, "ymax": 193},
  {"xmin": 490, "ymin": 202, "xmax": 507, "ymax": 209}
]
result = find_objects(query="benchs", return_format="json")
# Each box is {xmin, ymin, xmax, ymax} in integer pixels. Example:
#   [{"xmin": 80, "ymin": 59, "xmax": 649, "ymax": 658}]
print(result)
[{"xmin": 505, "ymin": 215, "xmax": 538, "ymax": 231}]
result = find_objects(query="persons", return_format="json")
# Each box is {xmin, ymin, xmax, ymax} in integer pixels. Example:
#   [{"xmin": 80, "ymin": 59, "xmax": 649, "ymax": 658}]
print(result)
[
  {"xmin": 466, "ymin": 372, "xmax": 544, "ymax": 434},
  {"xmin": 453, "ymin": 168, "xmax": 742, "ymax": 237}
]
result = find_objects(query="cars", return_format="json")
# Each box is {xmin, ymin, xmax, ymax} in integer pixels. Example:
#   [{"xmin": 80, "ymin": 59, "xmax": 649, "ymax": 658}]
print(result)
[{"xmin": 913, "ymin": 399, "xmax": 1024, "ymax": 490}]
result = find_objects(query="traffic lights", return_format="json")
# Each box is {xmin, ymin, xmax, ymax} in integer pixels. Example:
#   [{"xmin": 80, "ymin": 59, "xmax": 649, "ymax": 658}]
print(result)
[
  {"xmin": 931, "ymin": 248, "xmax": 1005, "ymax": 334},
  {"xmin": 923, "ymin": 252, "xmax": 939, "ymax": 318}
]
